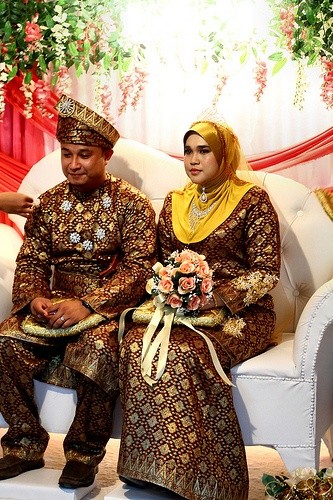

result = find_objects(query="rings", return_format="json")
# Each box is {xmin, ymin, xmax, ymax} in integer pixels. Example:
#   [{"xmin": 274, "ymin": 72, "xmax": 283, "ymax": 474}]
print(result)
[{"xmin": 59, "ymin": 316, "xmax": 65, "ymax": 322}]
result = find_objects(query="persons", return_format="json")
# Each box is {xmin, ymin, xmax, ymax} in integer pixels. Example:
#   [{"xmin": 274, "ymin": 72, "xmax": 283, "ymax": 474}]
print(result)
[
  {"xmin": 0, "ymin": 192, "xmax": 33, "ymax": 218},
  {"xmin": 116, "ymin": 119, "xmax": 283, "ymax": 500},
  {"xmin": 0, "ymin": 93, "xmax": 157, "ymax": 490}
]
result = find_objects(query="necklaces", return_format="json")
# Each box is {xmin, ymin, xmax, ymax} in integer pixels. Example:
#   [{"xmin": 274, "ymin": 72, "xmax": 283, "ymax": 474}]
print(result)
[{"xmin": 188, "ymin": 198, "xmax": 216, "ymax": 228}]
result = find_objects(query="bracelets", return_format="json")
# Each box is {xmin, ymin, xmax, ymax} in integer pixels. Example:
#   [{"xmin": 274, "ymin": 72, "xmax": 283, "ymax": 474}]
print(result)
[{"xmin": 212, "ymin": 294, "xmax": 218, "ymax": 309}]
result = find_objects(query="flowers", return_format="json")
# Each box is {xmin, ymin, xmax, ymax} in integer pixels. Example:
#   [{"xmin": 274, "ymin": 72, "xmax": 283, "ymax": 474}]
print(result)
[
  {"xmin": 0, "ymin": 0, "xmax": 333, "ymax": 120},
  {"xmin": 261, "ymin": 465, "xmax": 333, "ymax": 500},
  {"xmin": 145, "ymin": 249, "xmax": 216, "ymax": 312}
]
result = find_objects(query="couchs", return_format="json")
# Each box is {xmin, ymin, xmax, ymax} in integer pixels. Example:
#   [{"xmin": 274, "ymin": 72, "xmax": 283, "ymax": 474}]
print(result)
[{"xmin": 0, "ymin": 138, "xmax": 333, "ymax": 478}]
